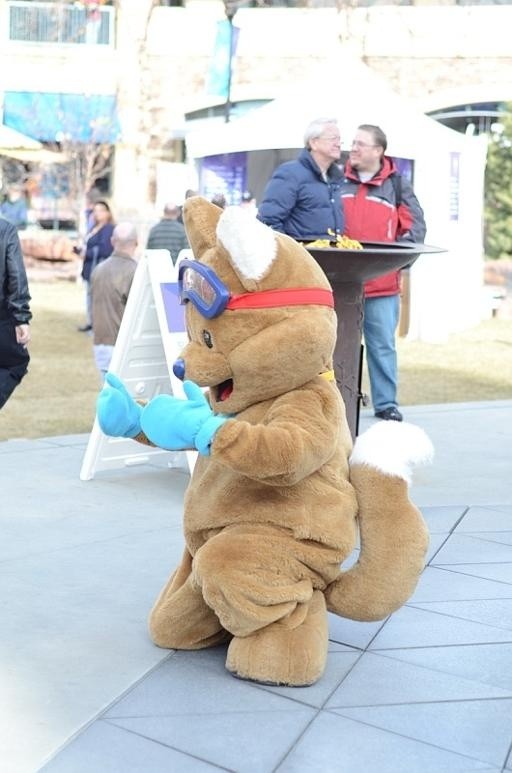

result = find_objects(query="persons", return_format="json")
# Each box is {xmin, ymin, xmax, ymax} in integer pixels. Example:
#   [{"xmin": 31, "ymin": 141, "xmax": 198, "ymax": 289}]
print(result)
[
  {"xmin": 0, "ymin": 217, "xmax": 33, "ymax": 414},
  {"xmin": 88, "ymin": 219, "xmax": 141, "ymax": 381},
  {"xmin": 141, "ymin": 190, "xmax": 230, "ymax": 264},
  {"xmin": 75, "ymin": 0, "xmax": 104, "ymax": 44},
  {"xmin": 1, "ymin": 183, "xmax": 31, "ymax": 232},
  {"xmin": 74, "ymin": 198, "xmax": 118, "ymax": 333},
  {"xmin": 338, "ymin": 123, "xmax": 429, "ymax": 421},
  {"xmin": 251, "ymin": 111, "xmax": 347, "ymax": 247}
]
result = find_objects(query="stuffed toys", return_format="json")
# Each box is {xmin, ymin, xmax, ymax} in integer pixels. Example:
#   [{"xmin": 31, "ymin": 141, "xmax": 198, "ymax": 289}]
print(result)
[{"xmin": 88, "ymin": 189, "xmax": 434, "ymax": 689}]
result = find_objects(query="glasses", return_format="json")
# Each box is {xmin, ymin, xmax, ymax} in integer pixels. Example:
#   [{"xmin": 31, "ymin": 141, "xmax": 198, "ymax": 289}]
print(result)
[
  {"xmin": 351, "ymin": 140, "xmax": 382, "ymax": 148},
  {"xmin": 315, "ymin": 134, "xmax": 344, "ymax": 145}
]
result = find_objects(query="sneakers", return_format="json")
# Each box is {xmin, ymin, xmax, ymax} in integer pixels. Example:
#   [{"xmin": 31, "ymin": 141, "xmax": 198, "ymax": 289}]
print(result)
[
  {"xmin": 78, "ymin": 324, "xmax": 93, "ymax": 332},
  {"xmin": 375, "ymin": 406, "xmax": 403, "ymax": 421}
]
